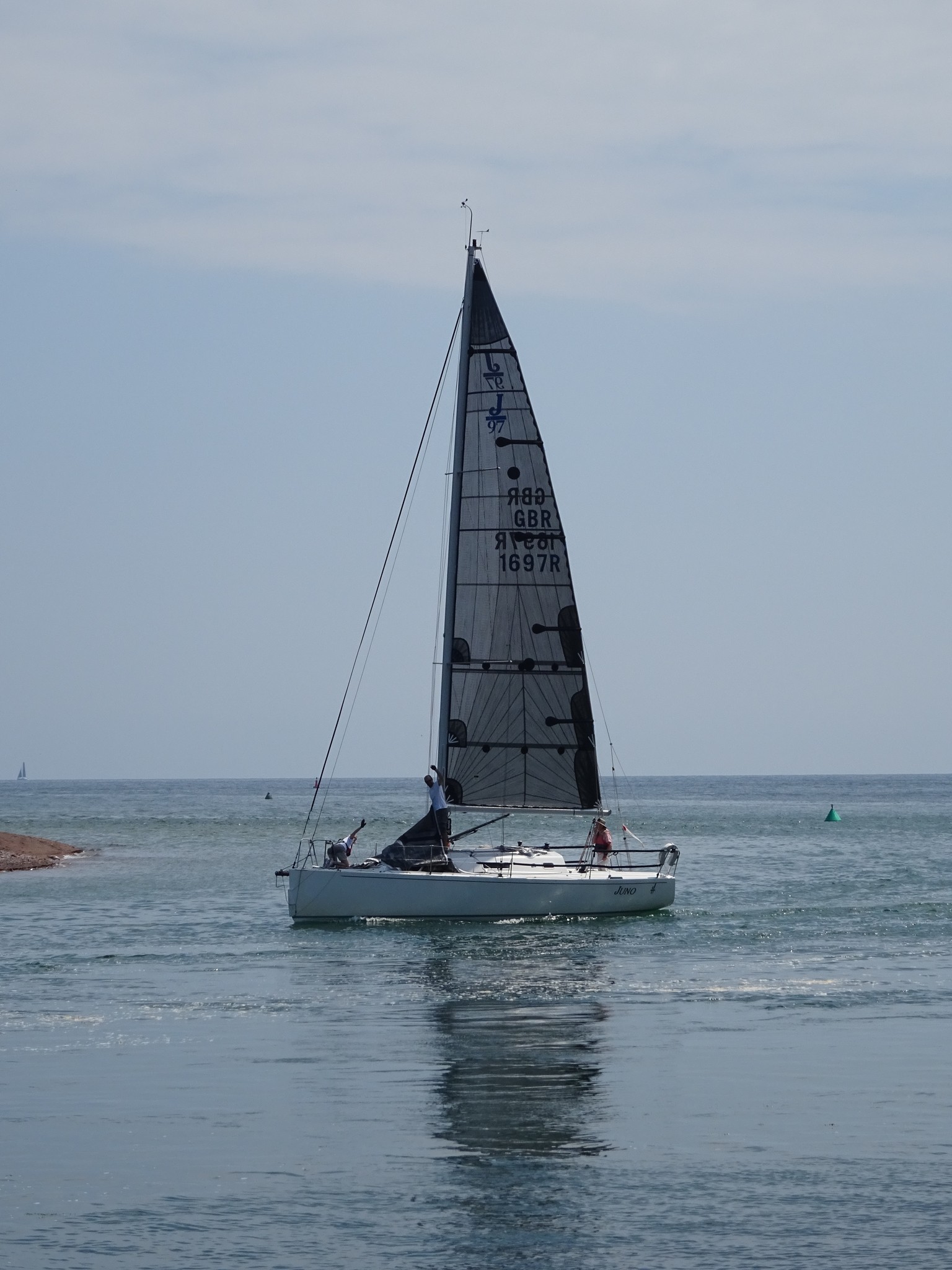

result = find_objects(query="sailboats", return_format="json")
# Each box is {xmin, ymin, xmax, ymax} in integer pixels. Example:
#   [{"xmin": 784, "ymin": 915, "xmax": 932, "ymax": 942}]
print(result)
[
  {"xmin": 16, "ymin": 762, "xmax": 30, "ymax": 780},
  {"xmin": 269, "ymin": 198, "xmax": 684, "ymax": 919}
]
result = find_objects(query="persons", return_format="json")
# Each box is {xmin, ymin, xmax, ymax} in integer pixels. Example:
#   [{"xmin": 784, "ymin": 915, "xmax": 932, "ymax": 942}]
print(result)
[
  {"xmin": 591, "ymin": 818, "xmax": 613, "ymax": 872},
  {"xmin": 322, "ymin": 819, "xmax": 367, "ymax": 869},
  {"xmin": 424, "ymin": 765, "xmax": 449, "ymax": 855}
]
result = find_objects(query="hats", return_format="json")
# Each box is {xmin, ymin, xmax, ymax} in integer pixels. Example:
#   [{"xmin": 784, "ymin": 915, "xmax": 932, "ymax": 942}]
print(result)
[{"xmin": 596, "ymin": 818, "xmax": 607, "ymax": 828}]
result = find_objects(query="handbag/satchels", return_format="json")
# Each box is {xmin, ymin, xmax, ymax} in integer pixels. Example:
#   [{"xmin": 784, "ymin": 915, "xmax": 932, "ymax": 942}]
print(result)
[
  {"xmin": 594, "ymin": 843, "xmax": 608, "ymax": 852},
  {"xmin": 339, "ymin": 841, "xmax": 351, "ymax": 856}
]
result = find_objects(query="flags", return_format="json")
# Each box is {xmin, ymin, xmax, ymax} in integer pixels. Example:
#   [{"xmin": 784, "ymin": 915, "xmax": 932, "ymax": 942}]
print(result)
[{"xmin": 622, "ymin": 824, "xmax": 644, "ymax": 848}]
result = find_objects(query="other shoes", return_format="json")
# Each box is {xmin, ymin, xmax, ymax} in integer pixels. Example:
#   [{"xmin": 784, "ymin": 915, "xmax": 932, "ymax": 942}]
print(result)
[{"xmin": 323, "ymin": 858, "xmax": 333, "ymax": 869}]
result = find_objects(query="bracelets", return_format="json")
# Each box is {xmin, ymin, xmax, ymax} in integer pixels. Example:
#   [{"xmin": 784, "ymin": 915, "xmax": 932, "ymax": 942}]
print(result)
[
  {"xmin": 604, "ymin": 852, "xmax": 608, "ymax": 856},
  {"xmin": 435, "ymin": 770, "xmax": 437, "ymax": 772}
]
